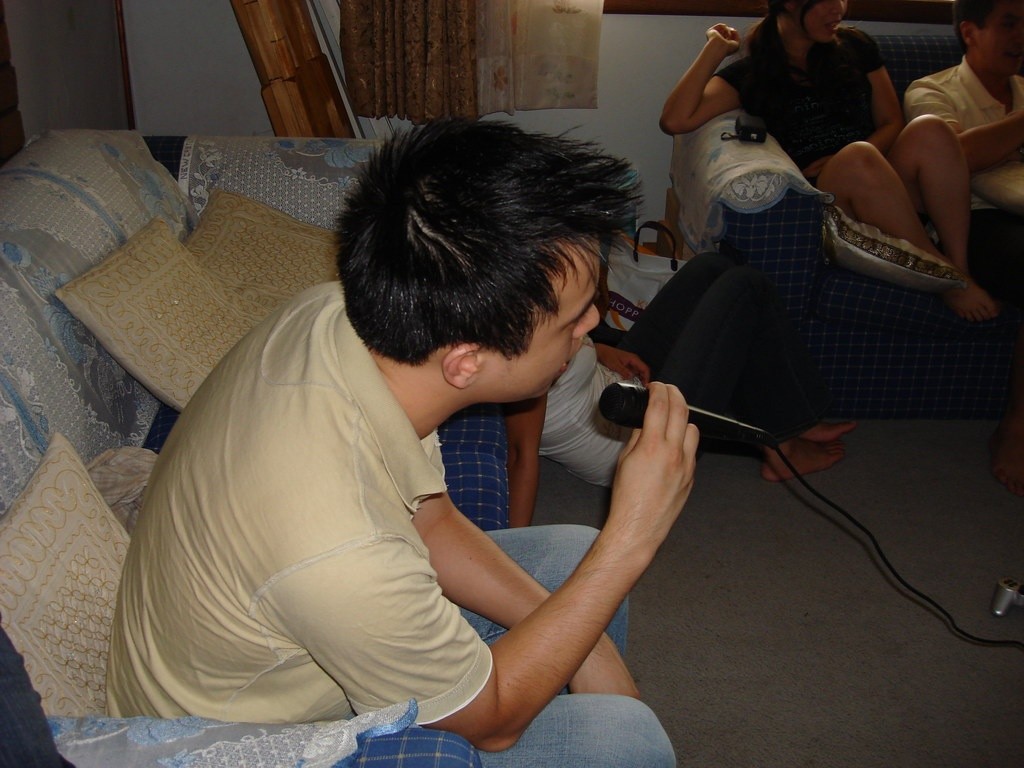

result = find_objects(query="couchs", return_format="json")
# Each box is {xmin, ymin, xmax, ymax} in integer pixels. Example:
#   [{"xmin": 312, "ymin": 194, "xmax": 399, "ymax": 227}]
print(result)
[
  {"xmin": 0, "ymin": 128, "xmax": 512, "ymax": 768},
  {"xmin": 668, "ymin": 34, "xmax": 1024, "ymax": 423}
]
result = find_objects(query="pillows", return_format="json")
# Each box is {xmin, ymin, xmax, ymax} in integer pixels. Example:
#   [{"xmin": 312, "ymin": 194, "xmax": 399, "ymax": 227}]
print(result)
[
  {"xmin": 52, "ymin": 215, "xmax": 273, "ymax": 413},
  {"xmin": 181, "ymin": 190, "xmax": 342, "ymax": 313},
  {"xmin": 0, "ymin": 430, "xmax": 132, "ymax": 717},
  {"xmin": 821, "ymin": 206, "xmax": 967, "ymax": 295},
  {"xmin": 971, "ymin": 161, "xmax": 1024, "ymax": 215}
]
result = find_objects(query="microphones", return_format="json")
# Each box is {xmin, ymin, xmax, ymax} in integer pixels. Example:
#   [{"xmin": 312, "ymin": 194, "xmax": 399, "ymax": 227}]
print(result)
[{"xmin": 598, "ymin": 382, "xmax": 778, "ymax": 449}]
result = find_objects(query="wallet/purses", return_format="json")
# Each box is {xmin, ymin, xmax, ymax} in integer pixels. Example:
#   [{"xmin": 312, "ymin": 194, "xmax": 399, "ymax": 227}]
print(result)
[{"xmin": 721, "ymin": 115, "xmax": 767, "ymax": 143}]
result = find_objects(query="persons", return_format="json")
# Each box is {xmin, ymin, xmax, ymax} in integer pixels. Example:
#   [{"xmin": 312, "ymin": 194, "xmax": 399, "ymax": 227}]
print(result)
[
  {"xmin": 106, "ymin": 117, "xmax": 699, "ymax": 767},
  {"xmin": 658, "ymin": 0, "xmax": 1000, "ymax": 322},
  {"xmin": 902, "ymin": 0, "xmax": 1024, "ymax": 301},
  {"xmin": 505, "ymin": 252, "xmax": 858, "ymax": 529}
]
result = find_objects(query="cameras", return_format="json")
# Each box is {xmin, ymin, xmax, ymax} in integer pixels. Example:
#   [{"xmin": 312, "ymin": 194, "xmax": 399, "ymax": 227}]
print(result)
[{"xmin": 735, "ymin": 115, "xmax": 766, "ymax": 141}]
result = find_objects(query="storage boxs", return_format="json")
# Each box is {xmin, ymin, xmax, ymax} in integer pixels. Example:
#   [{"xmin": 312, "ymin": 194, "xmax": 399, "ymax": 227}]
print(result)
[{"xmin": 658, "ymin": 189, "xmax": 683, "ymax": 260}]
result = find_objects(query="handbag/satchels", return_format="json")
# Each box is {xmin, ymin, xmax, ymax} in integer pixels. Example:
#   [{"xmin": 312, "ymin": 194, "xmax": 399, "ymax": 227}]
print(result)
[{"xmin": 601, "ymin": 221, "xmax": 689, "ymax": 331}]
